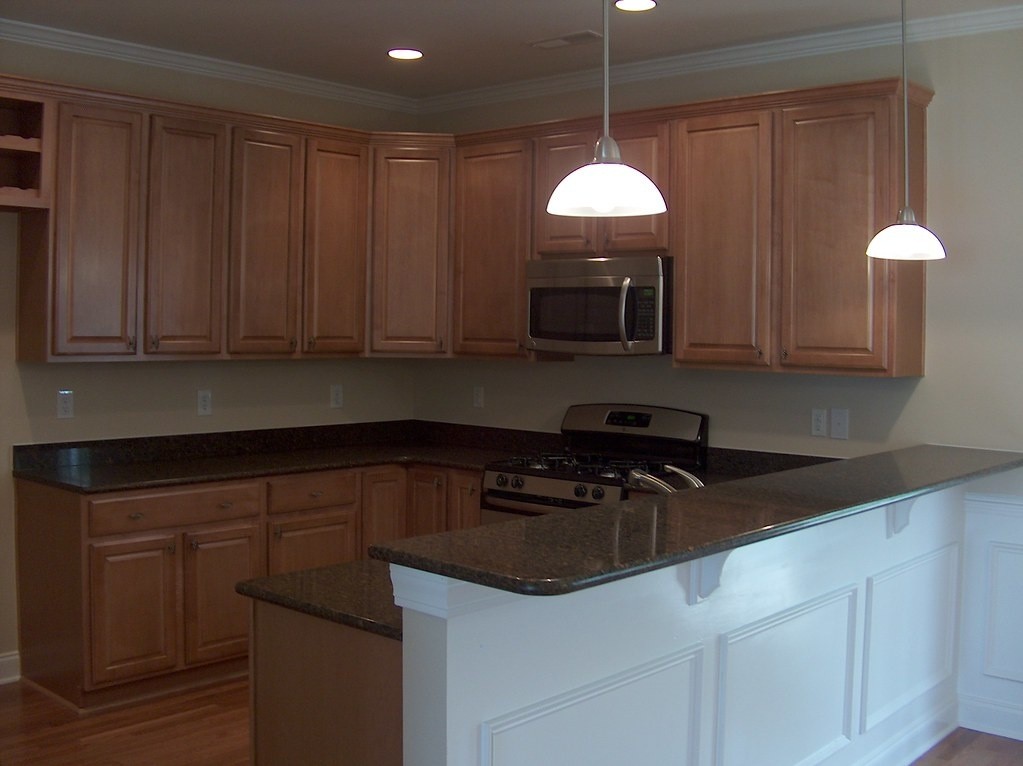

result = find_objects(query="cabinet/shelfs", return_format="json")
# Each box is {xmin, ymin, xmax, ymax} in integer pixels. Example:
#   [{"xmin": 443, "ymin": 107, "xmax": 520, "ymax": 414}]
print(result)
[
  {"xmin": 453, "ymin": 126, "xmax": 575, "ymax": 363},
  {"xmin": 14, "ymin": 477, "xmax": 267, "ymax": 720},
  {"xmin": 405, "ymin": 462, "xmax": 447, "ymax": 539},
  {"xmin": 368, "ymin": 130, "xmax": 458, "ymax": 359},
  {"xmin": 530, "ymin": 105, "xmax": 673, "ymax": 260},
  {"xmin": 15, "ymin": 82, "xmax": 224, "ymax": 367},
  {"xmin": 223, "ymin": 109, "xmax": 370, "ymax": 362},
  {"xmin": 445, "ymin": 466, "xmax": 484, "ymax": 532},
  {"xmin": 358, "ymin": 464, "xmax": 408, "ymax": 559},
  {"xmin": 672, "ymin": 74, "xmax": 936, "ymax": 379},
  {"xmin": 265, "ymin": 467, "xmax": 361, "ymax": 577}
]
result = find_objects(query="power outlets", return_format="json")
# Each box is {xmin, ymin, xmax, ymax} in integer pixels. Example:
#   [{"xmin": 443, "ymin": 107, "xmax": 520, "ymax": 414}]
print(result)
[
  {"xmin": 830, "ymin": 407, "xmax": 849, "ymax": 440},
  {"xmin": 197, "ymin": 389, "xmax": 213, "ymax": 417},
  {"xmin": 810, "ymin": 407, "xmax": 828, "ymax": 438},
  {"xmin": 329, "ymin": 383, "xmax": 344, "ymax": 409},
  {"xmin": 55, "ymin": 390, "xmax": 75, "ymax": 419},
  {"xmin": 472, "ymin": 385, "xmax": 485, "ymax": 409}
]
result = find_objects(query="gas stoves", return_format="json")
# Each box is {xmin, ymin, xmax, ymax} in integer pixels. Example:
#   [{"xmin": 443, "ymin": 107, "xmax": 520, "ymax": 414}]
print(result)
[{"xmin": 481, "ymin": 403, "xmax": 709, "ymax": 487}]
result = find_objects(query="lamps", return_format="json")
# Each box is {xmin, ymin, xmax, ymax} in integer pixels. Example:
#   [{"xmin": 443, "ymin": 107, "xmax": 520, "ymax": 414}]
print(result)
[
  {"xmin": 864, "ymin": 0, "xmax": 946, "ymax": 261},
  {"xmin": 546, "ymin": 0, "xmax": 667, "ymax": 217}
]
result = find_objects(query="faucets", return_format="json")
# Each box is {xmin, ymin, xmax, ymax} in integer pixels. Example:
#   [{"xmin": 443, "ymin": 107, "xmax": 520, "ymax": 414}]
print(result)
[{"xmin": 629, "ymin": 463, "xmax": 705, "ymax": 494}]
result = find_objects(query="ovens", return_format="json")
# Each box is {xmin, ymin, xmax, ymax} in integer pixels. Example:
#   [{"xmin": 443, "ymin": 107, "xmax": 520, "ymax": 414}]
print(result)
[{"xmin": 479, "ymin": 471, "xmax": 629, "ymax": 526}]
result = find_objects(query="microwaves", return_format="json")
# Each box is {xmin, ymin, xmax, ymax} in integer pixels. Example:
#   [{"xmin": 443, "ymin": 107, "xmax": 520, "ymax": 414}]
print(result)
[{"xmin": 524, "ymin": 255, "xmax": 671, "ymax": 355}]
